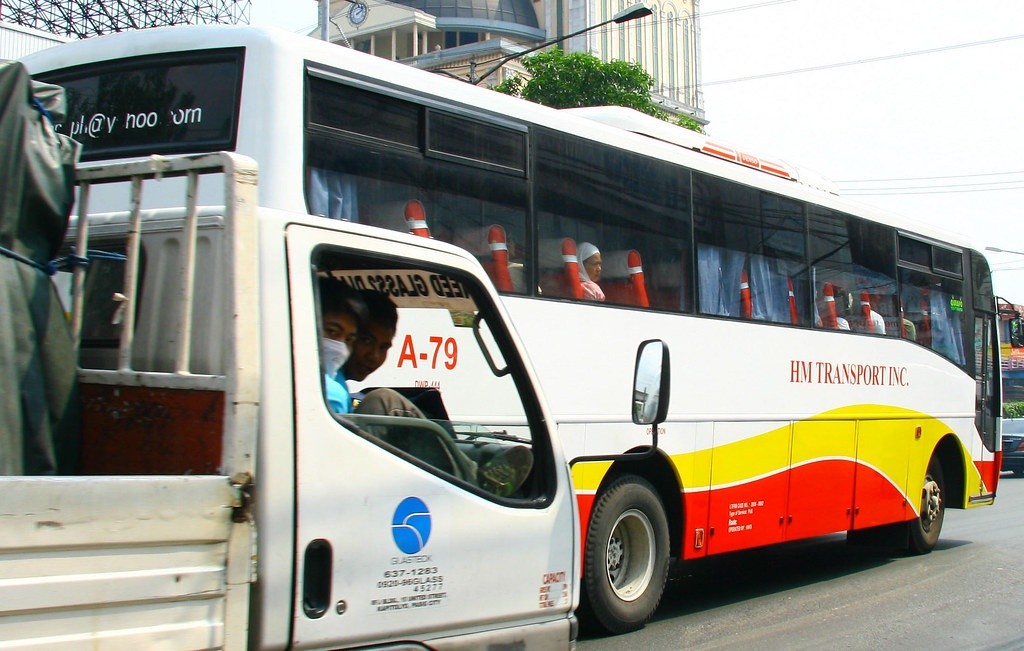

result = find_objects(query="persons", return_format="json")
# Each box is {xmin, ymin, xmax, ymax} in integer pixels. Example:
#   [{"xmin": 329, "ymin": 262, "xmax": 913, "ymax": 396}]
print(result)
[
  {"xmin": 835, "ymin": 294, "xmax": 851, "ymax": 330},
  {"xmin": 319, "ymin": 274, "xmax": 534, "ymax": 496},
  {"xmin": 815, "ymin": 287, "xmax": 823, "ymax": 328},
  {"xmin": 576, "ymin": 241, "xmax": 605, "ymax": 302},
  {"xmin": 869, "ymin": 300, "xmax": 886, "ymax": 334},
  {"xmin": 901, "ymin": 302, "xmax": 918, "ymax": 342}
]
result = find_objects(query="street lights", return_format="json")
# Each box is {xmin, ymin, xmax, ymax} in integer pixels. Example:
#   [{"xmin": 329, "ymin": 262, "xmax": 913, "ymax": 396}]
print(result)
[{"xmin": 420, "ymin": 3, "xmax": 655, "ymax": 237}]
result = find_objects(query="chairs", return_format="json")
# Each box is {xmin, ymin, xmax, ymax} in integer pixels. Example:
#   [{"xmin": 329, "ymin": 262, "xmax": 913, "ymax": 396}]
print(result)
[
  {"xmin": 453, "ymin": 226, "xmax": 518, "ymax": 299},
  {"xmin": 373, "ymin": 195, "xmax": 440, "ymax": 239},
  {"xmin": 652, "ymin": 261, "xmax": 933, "ymax": 351},
  {"xmin": 536, "ymin": 237, "xmax": 654, "ymax": 309}
]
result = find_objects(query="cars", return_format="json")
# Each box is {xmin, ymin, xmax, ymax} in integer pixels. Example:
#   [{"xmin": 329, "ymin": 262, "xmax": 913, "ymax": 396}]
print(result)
[{"xmin": 1002, "ymin": 417, "xmax": 1024, "ymax": 477}]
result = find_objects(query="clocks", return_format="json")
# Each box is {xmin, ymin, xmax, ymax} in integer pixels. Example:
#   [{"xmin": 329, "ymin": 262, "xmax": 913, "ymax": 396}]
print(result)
[{"xmin": 344, "ymin": 0, "xmax": 371, "ymax": 30}]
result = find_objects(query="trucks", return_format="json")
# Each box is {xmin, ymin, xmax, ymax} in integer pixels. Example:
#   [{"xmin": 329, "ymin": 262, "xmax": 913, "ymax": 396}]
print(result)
[{"xmin": 0, "ymin": 149, "xmax": 674, "ymax": 651}]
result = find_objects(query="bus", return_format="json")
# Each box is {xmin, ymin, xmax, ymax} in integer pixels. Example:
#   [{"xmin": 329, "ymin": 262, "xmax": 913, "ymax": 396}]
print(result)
[{"xmin": 12, "ymin": 21, "xmax": 1024, "ymax": 634}]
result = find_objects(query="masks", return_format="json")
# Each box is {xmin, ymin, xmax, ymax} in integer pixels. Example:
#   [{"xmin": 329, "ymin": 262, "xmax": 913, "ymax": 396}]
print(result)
[{"xmin": 323, "ymin": 338, "xmax": 350, "ymax": 376}]
